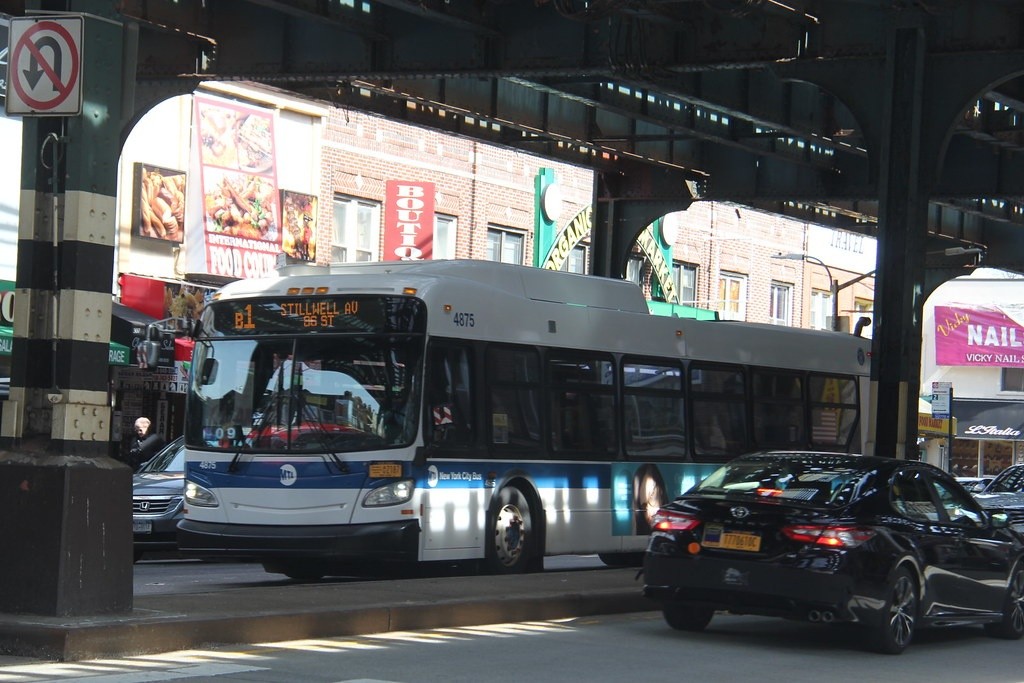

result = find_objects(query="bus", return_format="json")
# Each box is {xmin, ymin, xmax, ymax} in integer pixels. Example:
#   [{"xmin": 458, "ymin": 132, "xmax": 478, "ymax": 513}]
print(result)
[{"xmin": 174, "ymin": 260, "xmax": 872, "ymax": 585}]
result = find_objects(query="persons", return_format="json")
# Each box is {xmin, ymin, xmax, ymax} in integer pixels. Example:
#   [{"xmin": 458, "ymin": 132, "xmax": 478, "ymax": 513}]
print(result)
[{"xmin": 128, "ymin": 415, "xmax": 168, "ymax": 474}]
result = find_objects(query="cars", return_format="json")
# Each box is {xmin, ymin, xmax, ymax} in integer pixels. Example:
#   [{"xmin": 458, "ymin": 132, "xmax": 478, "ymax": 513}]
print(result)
[
  {"xmin": 941, "ymin": 463, "xmax": 1024, "ymax": 534},
  {"xmin": 130, "ymin": 425, "xmax": 287, "ymax": 564},
  {"xmin": 635, "ymin": 449, "xmax": 1024, "ymax": 654}
]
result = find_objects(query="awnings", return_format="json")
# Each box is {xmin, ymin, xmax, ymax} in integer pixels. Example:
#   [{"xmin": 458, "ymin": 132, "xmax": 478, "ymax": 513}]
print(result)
[
  {"xmin": 110, "ymin": 297, "xmax": 175, "ymax": 368},
  {"xmin": 0, "ymin": 326, "xmax": 130, "ymax": 367}
]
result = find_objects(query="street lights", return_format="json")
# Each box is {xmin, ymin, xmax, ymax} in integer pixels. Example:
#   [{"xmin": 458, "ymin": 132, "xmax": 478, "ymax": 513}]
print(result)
[{"xmin": 770, "ymin": 251, "xmax": 876, "ymax": 332}]
